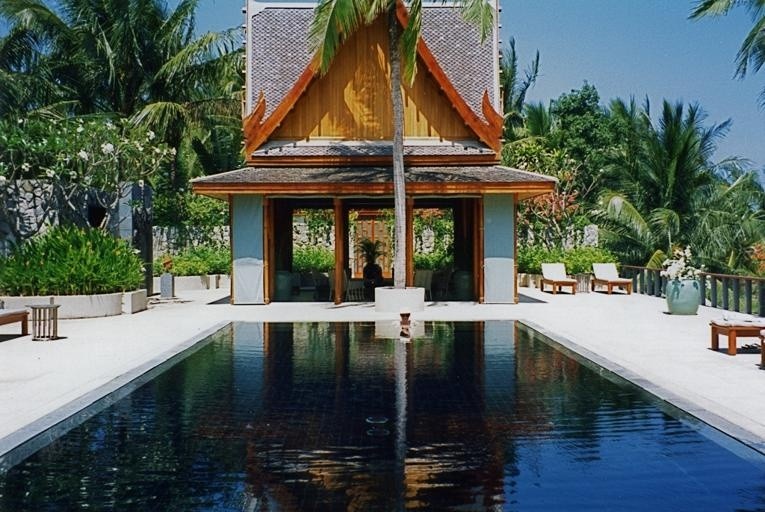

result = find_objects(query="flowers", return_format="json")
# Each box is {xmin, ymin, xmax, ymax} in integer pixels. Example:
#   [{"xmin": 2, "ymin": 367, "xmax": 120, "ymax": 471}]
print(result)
[{"xmin": 659, "ymin": 243, "xmax": 713, "ymax": 283}]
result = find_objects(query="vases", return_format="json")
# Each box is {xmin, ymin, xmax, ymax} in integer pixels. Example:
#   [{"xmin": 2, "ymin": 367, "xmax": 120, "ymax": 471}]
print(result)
[{"xmin": 664, "ymin": 278, "xmax": 701, "ymax": 315}]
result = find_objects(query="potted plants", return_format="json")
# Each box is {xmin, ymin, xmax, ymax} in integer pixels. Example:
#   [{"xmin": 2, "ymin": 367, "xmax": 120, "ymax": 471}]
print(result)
[{"xmin": 352, "ymin": 238, "xmax": 386, "ymax": 294}]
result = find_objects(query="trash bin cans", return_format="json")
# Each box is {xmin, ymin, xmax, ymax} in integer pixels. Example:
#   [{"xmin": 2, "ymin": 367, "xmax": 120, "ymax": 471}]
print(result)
[{"xmin": 161, "ymin": 273, "xmax": 174, "ymax": 298}]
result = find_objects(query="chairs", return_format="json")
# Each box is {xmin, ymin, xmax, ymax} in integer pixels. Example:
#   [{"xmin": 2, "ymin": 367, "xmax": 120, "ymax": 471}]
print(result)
[
  {"xmin": 325, "ymin": 265, "xmax": 453, "ymax": 302},
  {"xmin": 539, "ymin": 261, "xmax": 633, "ymax": 295}
]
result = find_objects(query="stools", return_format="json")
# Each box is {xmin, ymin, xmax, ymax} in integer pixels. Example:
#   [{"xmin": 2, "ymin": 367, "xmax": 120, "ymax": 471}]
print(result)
[
  {"xmin": 0, "ymin": 307, "xmax": 30, "ymax": 336},
  {"xmin": 709, "ymin": 317, "xmax": 765, "ymax": 355}
]
